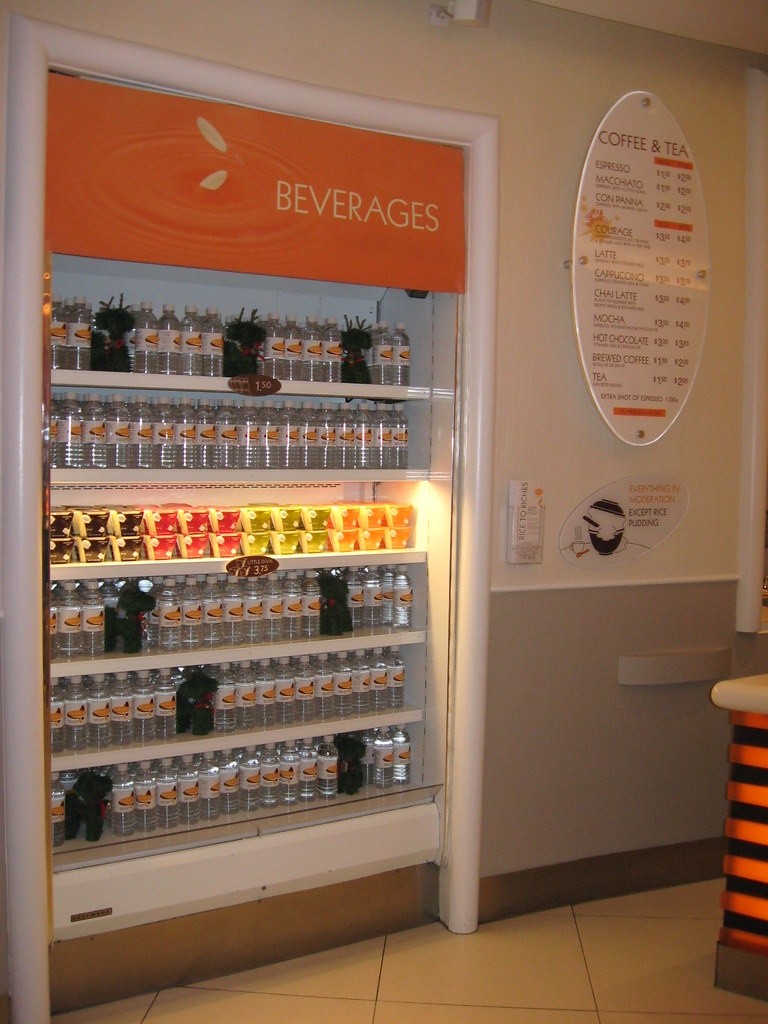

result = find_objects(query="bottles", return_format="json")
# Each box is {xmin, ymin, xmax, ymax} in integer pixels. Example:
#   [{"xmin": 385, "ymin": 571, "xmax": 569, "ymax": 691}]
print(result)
[
  {"xmin": 46, "ymin": 563, "xmax": 412, "ymax": 845},
  {"xmin": 43, "ymin": 293, "xmax": 411, "ymax": 468}
]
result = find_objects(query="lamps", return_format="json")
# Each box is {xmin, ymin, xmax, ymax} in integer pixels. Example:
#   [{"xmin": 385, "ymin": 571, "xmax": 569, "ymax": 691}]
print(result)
[{"xmin": 428, "ymin": 0, "xmax": 481, "ymax": 25}]
